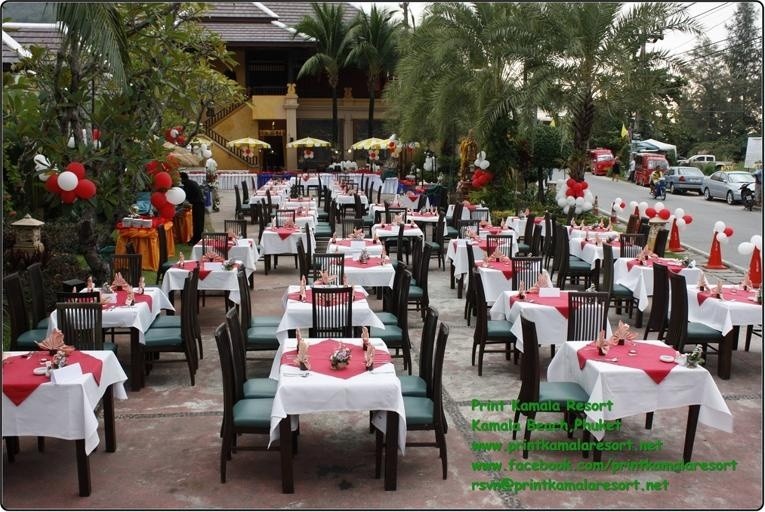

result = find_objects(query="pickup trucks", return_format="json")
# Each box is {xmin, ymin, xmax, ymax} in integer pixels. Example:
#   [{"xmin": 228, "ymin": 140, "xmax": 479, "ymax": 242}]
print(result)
[{"xmin": 679, "ymin": 154, "xmax": 732, "ymax": 171}]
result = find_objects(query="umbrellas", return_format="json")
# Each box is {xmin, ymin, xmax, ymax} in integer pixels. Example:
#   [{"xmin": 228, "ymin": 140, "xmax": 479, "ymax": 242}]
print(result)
[
  {"xmin": 225, "ymin": 137, "xmax": 271, "ymax": 149},
  {"xmin": 351, "ymin": 137, "xmax": 390, "ymax": 150},
  {"xmin": 286, "ymin": 137, "xmax": 332, "ymax": 148}
]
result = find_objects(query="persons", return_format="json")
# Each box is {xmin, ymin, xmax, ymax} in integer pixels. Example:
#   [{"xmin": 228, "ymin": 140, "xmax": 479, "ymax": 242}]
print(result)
[
  {"xmin": 627, "ymin": 158, "xmax": 635, "ymax": 183},
  {"xmin": 650, "ymin": 165, "xmax": 664, "ymax": 196},
  {"xmin": 179, "ymin": 171, "xmax": 206, "ymax": 246},
  {"xmin": 611, "ymin": 156, "xmax": 620, "ymax": 183}
]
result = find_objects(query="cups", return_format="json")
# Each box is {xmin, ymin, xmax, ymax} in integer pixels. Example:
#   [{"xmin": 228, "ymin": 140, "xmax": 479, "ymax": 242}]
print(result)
[
  {"xmin": 332, "ymin": 239, "xmax": 336, "ymax": 244},
  {"xmin": 571, "ymin": 224, "xmax": 610, "ymax": 232},
  {"xmin": 411, "ymin": 224, "xmax": 414, "ymax": 228},
  {"xmin": 297, "ymin": 339, "xmax": 374, "ymax": 377},
  {"xmin": 208, "ymin": 257, "xmax": 214, "ymax": 262},
  {"xmin": 373, "ymin": 240, "xmax": 376, "ymax": 244},
  {"xmin": 228, "ymin": 237, "xmax": 233, "ymax": 241},
  {"xmin": 299, "ymin": 281, "xmax": 355, "ymax": 306},
  {"xmin": 71, "ymin": 285, "xmax": 145, "ymax": 306},
  {"xmin": 644, "ymin": 255, "xmax": 648, "ymax": 260},
  {"xmin": 618, "ymin": 338, "xmax": 624, "ymax": 345},
  {"xmin": 48, "ymin": 349, "xmax": 57, "ymax": 355},
  {"xmin": 700, "ymin": 285, "xmax": 762, "ymax": 304},
  {"xmin": 628, "ymin": 342, "xmax": 640, "ymax": 357},
  {"xmin": 496, "ymin": 258, "xmax": 499, "ymax": 262},
  {"xmin": 381, "ymin": 224, "xmax": 385, "ymax": 228},
  {"xmin": 598, "ymin": 347, "xmax": 606, "ymax": 356},
  {"xmin": 519, "ymin": 291, "xmax": 525, "ymax": 300},
  {"xmin": 639, "ymin": 260, "xmax": 643, "ymax": 265}
]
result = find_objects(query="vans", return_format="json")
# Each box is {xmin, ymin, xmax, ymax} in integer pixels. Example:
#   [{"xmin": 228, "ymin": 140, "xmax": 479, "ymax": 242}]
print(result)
[{"xmin": 634, "ymin": 152, "xmax": 669, "ymax": 188}]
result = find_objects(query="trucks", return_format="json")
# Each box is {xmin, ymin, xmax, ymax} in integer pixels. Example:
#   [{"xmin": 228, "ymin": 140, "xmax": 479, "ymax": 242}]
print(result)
[{"xmin": 587, "ymin": 147, "xmax": 616, "ymax": 177}]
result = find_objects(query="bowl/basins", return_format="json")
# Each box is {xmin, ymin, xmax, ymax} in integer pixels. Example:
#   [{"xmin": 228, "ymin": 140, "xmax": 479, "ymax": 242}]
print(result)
[{"xmin": 122, "ymin": 217, "xmax": 152, "ymax": 228}]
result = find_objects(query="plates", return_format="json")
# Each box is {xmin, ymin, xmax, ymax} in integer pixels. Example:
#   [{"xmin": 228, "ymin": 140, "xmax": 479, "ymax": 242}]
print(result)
[{"xmin": 660, "ymin": 355, "xmax": 675, "ymax": 363}]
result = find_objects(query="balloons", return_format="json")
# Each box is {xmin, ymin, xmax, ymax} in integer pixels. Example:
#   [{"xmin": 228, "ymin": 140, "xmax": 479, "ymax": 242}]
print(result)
[
  {"xmin": 303, "ymin": 148, "xmax": 315, "ymax": 159},
  {"xmin": 612, "ymin": 198, "xmax": 763, "ymax": 259},
  {"xmin": 243, "ymin": 146, "xmax": 255, "ymax": 158},
  {"xmin": 33, "ymin": 125, "xmax": 102, "ymax": 206},
  {"xmin": 555, "ymin": 176, "xmax": 595, "ymax": 215},
  {"xmin": 142, "ymin": 125, "xmax": 218, "ymax": 222},
  {"xmin": 367, "ymin": 134, "xmax": 421, "ymax": 161},
  {"xmin": 423, "ymin": 153, "xmax": 442, "ymax": 173},
  {"xmin": 471, "ymin": 150, "xmax": 492, "ymax": 191}
]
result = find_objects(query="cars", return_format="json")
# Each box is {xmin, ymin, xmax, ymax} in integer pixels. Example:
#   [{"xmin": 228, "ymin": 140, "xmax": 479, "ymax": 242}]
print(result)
[
  {"xmin": 663, "ymin": 167, "xmax": 706, "ymax": 195},
  {"xmin": 702, "ymin": 170, "xmax": 757, "ymax": 202}
]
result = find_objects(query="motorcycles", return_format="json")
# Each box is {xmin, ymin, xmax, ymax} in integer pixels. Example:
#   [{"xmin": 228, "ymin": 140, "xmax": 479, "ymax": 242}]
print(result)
[
  {"xmin": 649, "ymin": 178, "xmax": 670, "ymax": 201},
  {"xmin": 736, "ymin": 182, "xmax": 756, "ymax": 212}
]
